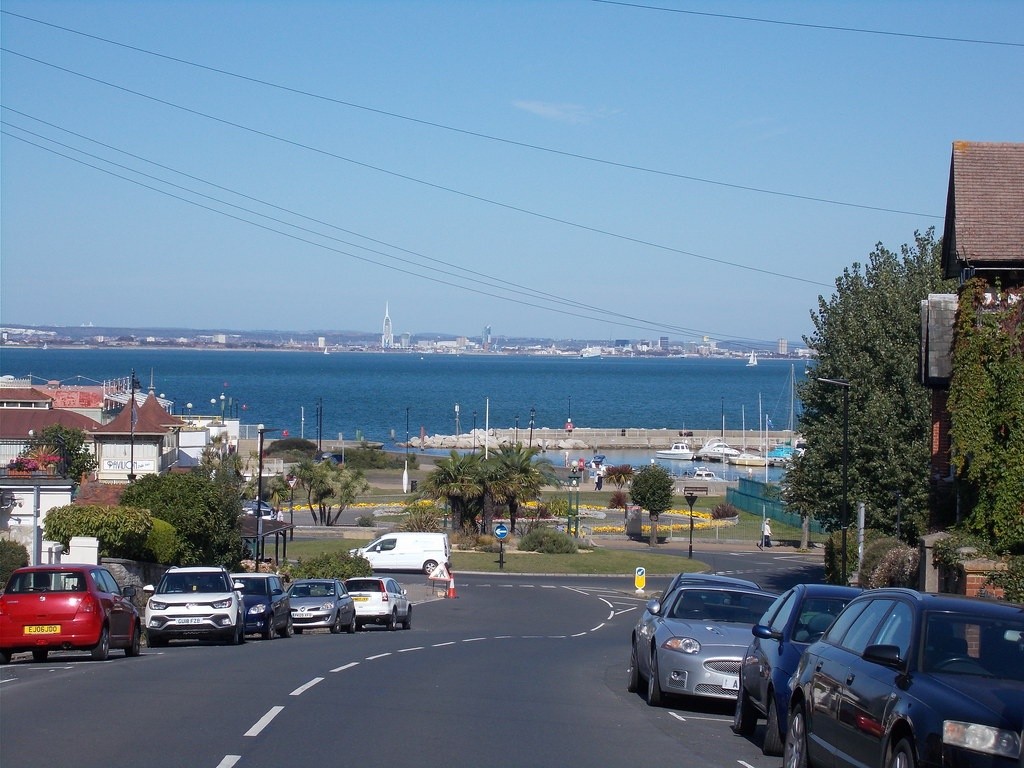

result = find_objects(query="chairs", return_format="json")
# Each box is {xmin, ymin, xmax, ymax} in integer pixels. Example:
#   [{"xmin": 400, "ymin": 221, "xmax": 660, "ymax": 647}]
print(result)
[
  {"xmin": 680, "ymin": 596, "xmax": 705, "ymax": 619},
  {"xmin": 796, "ymin": 614, "xmax": 834, "ymax": 642}
]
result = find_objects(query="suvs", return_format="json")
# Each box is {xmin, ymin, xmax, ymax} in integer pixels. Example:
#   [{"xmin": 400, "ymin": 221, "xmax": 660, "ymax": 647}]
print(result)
[
  {"xmin": 230, "ymin": 570, "xmax": 294, "ymax": 640},
  {"xmin": 732, "ymin": 583, "xmax": 970, "ymax": 756},
  {"xmin": 140, "ymin": 565, "xmax": 249, "ymax": 647},
  {"xmin": 778, "ymin": 587, "xmax": 1024, "ymax": 768}
]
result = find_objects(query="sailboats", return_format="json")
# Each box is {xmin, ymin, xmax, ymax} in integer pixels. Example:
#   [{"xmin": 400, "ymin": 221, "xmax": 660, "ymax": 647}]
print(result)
[{"xmin": 745, "ymin": 349, "xmax": 758, "ymax": 367}]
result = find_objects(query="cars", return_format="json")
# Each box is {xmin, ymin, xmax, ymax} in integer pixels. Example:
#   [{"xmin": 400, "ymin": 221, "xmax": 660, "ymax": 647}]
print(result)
[
  {"xmin": 0, "ymin": 562, "xmax": 142, "ymax": 665},
  {"xmin": 625, "ymin": 584, "xmax": 804, "ymax": 710},
  {"xmin": 242, "ymin": 499, "xmax": 276, "ymax": 520},
  {"xmin": 656, "ymin": 571, "xmax": 765, "ymax": 612},
  {"xmin": 315, "ymin": 452, "xmax": 346, "ymax": 466},
  {"xmin": 286, "ymin": 578, "xmax": 359, "ymax": 635},
  {"xmin": 343, "ymin": 575, "xmax": 414, "ymax": 631}
]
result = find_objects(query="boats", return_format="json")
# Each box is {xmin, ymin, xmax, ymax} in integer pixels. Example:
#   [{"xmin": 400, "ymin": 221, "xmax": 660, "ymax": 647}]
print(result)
[
  {"xmin": 571, "ymin": 454, "xmax": 616, "ymax": 479},
  {"xmin": 686, "ymin": 469, "xmax": 728, "ymax": 481},
  {"xmin": 652, "ymin": 362, "xmax": 809, "ymax": 469}
]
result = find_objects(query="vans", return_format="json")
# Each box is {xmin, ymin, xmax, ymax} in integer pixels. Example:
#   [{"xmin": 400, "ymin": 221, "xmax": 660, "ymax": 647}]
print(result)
[{"xmin": 348, "ymin": 530, "xmax": 453, "ymax": 576}]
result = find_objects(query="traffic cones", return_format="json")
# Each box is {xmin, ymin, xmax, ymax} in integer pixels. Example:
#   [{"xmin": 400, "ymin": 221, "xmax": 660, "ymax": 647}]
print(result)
[{"xmin": 446, "ymin": 577, "xmax": 458, "ymax": 599}]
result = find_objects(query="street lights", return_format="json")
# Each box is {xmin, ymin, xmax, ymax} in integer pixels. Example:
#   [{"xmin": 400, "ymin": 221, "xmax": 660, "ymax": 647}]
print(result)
[
  {"xmin": 210, "ymin": 398, "xmax": 217, "ymax": 426},
  {"xmin": 528, "ymin": 407, "xmax": 536, "ymax": 448},
  {"xmin": 186, "ymin": 402, "xmax": 193, "ymax": 430},
  {"xmin": 126, "ymin": 367, "xmax": 143, "ymax": 484},
  {"xmin": 515, "ymin": 415, "xmax": 520, "ymax": 444},
  {"xmin": 220, "ymin": 394, "xmax": 226, "ymax": 426},
  {"xmin": 473, "ymin": 410, "xmax": 478, "ymax": 456},
  {"xmin": 816, "ymin": 376, "xmax": 854, "ymax": 587}
]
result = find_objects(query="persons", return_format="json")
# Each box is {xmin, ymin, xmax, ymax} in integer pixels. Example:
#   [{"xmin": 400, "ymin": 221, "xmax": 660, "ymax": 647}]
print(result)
[
  {"xmin": 594, "ymin": 473, "xmax": 604, "ymax": 491},
  {"xmin": 760, "ymin": 518, "xmax": 773, "ymax": 548},
  {"xmin": 270, "ymin": 506, "xmax": 283, "ymax": 522}
]
